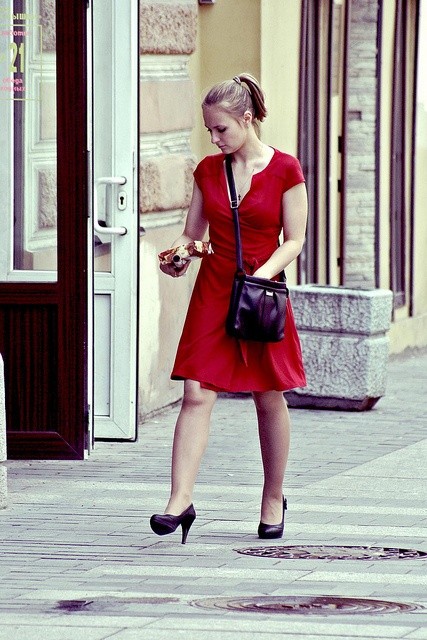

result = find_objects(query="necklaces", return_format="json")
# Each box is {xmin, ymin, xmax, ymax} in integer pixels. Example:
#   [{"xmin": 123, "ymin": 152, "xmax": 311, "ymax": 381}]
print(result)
[{"xmin": 232, "ymin": 144, "xmax": 265, "ymax": 201}]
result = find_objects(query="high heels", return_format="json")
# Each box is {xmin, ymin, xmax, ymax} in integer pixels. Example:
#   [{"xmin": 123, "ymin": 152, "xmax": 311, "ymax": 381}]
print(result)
[
  {"xmin": 150, "ymin": 503, "xmax": 196, "ymax": 544},
  {"xmin": 258, "ymin": 494, "xmax": 287, "ymax": 539}
]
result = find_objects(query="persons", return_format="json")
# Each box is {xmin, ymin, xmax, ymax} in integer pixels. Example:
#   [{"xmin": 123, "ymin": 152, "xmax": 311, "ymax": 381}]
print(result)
[{"xmin": 148, "ymin": 73, "xmax": 308, "ymax": 543}]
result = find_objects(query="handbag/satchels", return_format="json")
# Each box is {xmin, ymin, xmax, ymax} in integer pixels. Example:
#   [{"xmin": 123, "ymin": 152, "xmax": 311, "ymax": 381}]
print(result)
[{"xmin": 224, "ymin": 154, "xmax": 289, "ymax": 342}]
